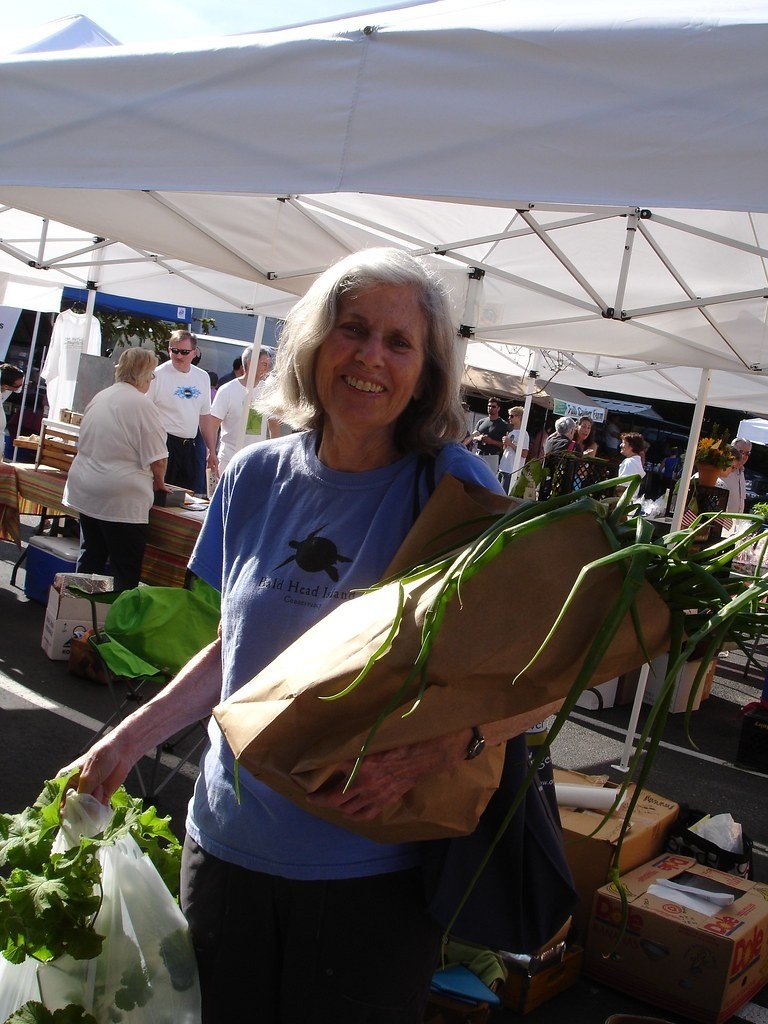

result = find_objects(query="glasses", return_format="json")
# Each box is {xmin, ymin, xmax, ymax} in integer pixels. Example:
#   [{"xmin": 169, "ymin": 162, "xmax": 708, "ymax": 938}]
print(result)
[
  {"xmin": 171, "ymin": 346, "xmax": 193, "ymax": 355},
  {"xmin": 488, "ymin": 405, "xmax": 497, "ymax": 408},
  {"xmin": 738, "ymin": 450, "xmax": 752, "ymax": 456},
  {"xmin": 508, "ymin": 414, "xmax": 518, "ymax": 418}
]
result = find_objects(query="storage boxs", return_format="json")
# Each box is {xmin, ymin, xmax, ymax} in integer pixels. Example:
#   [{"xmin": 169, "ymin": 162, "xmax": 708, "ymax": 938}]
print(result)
[
  {"xmin": 643, "ymin": 654, "xmax": 713, "ymax": 713},
  {"xmin": 42, "ymin": 586, "xmax": 113, "ymax": 660},
  {"xmin": 23, "ymin": 536, "xmax": 85, "ymax": 601},
  {"xmin": 575, "ymin": 675, "xmax": 619, "ymax": 710},
  {"xmin": 551, "ymin": 762, "xmax": 679, "ymax": 908},
  {"xmin": 590, "ymin": 853, "xmax": 768, "ymax": 1024},
  {"xmin": 701, "ymin": 655, "xmax": 718, "ymax": 701}
]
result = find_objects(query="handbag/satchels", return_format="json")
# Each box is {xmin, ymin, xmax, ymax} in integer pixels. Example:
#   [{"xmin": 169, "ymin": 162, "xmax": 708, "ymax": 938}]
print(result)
[
  {"xmin": 0, "ymin": 789, "xmax": 205, "ymax": 1024},
  {"xmin": 664, "ymin": 802, "xmax": 755, "ymax": 881},
  {"xmin": 211, "ymin": 506, "xmax": 738, "ymax": 837},
  {"xmin": 419, "ymin": 727, "xmax": 581, "ymax": 953}
]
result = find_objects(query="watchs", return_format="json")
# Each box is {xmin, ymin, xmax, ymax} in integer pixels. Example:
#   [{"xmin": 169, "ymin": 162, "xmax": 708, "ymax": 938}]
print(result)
[{"xmin": 465, "ymin": 727, "xmax": 486, "ymax": 760}]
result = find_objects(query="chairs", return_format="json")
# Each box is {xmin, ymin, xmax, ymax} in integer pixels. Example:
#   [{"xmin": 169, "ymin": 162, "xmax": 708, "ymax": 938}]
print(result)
[{"xmin": 1, "ymin": 461, "xmax": 75, "ymax": 586}]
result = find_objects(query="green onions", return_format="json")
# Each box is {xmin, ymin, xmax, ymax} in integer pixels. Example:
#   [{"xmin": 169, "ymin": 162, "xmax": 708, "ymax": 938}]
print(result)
[{"xmin": 320, "ymin": 475, "xmax": 768, "ymax": 966}]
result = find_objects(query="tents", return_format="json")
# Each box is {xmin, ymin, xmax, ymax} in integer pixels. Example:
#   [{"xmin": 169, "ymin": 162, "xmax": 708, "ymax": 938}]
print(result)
[{"xmin": 0, "ymin": 1, "xmax": 767, "ymax": 770}]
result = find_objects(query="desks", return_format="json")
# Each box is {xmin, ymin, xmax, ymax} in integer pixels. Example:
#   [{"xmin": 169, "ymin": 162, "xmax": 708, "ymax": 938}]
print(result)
[{"xmin": 5, "ymin": 462, "xmax": 211, "ymax": 590}]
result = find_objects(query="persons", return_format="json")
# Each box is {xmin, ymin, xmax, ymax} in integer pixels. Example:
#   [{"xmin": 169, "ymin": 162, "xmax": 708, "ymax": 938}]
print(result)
[
  {"xmin": 0, "ymin": 364, "xmax": 23, "ymax": 462},
  {"xmin": 691, "ymin": 438, "xmax": 752, "ymax": 579},
  {"xmin": 539, "ymin": 416, "xmax": 598, "ymax": 500},
  {"xmin": 209, "ymin": 346, "xmax": 280, "ymax": 479},
  {"xmin": 57, "ymin": 245, "xmax": 566, "ymax": 1024},
  {"xmin": 62, "ymin": 347, "xmax": 173, "ymax": 591},
  {"xmin": 615, "ymin": 433, "xmax": 679, "ymax": 498},
  {"xmin": 145, "ymin": 329, "xmax": 218, "ymax": 490},
  {"xmin": 461, "ymin": 396, "xmax": 529, "ymax": 491}
]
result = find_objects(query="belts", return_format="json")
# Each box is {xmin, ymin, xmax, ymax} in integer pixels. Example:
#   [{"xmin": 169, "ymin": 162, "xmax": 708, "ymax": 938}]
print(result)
[{"xmin": 168, "ymin": 433, "xmax": 197, "ymax": 447}]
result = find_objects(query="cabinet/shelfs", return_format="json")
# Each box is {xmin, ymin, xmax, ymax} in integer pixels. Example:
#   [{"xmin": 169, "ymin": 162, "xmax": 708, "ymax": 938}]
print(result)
[{"xmin": 34, "ymin": 418, "xmax": 82, "ymax": 473}]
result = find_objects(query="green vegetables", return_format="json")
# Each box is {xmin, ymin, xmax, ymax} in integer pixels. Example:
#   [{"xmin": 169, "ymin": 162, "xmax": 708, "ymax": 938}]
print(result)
[{"xmin": 0, "ymin": 768, "xmax": 200, "ymax": 1024}]
